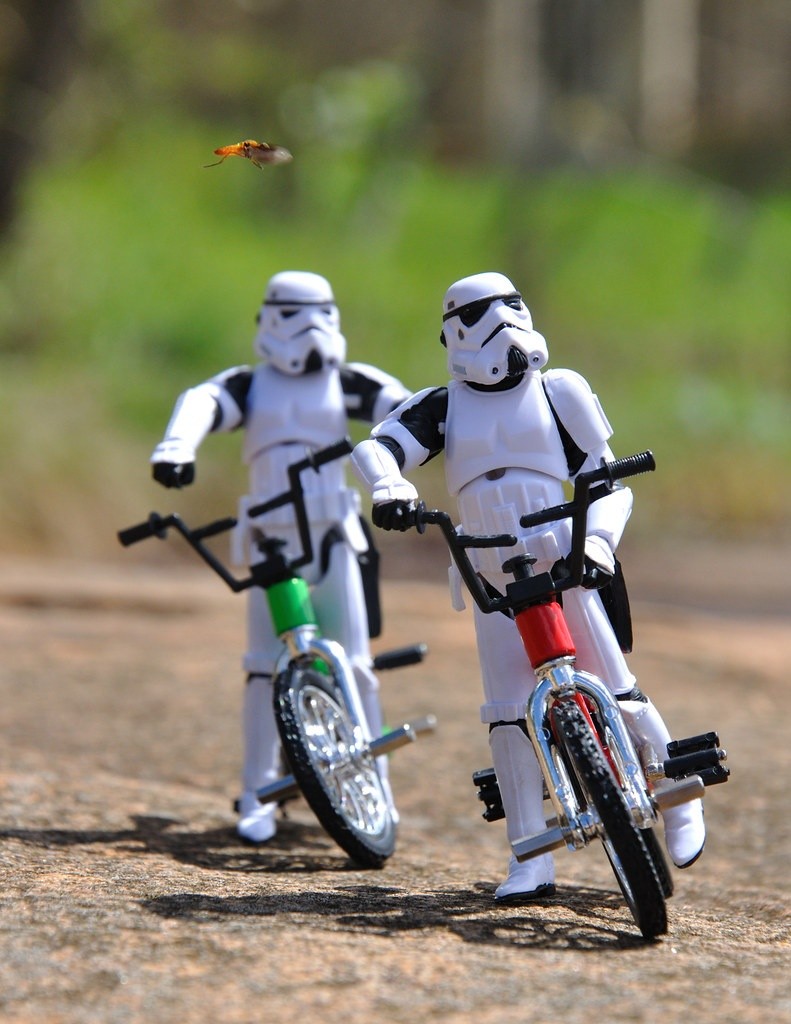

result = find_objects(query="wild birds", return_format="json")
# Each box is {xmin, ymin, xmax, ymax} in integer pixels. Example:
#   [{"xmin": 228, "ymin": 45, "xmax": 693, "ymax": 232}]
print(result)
[{"xmin": 203, "ymin": 139, "xmax": 292, "ymax": 171}]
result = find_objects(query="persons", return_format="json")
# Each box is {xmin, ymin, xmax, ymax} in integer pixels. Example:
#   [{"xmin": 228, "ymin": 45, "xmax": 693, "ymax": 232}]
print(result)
[
  {"xmin": 151, "ymin": 270, "xmax": 413, "ymax": 843},
  {"xmin": 351, "ymin": 272, "xmax": 707, "ymax": 901}
]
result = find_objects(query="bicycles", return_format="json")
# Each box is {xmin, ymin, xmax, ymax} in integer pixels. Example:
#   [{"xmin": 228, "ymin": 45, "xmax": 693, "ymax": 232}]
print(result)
[
  {"xmin": 397, "ymin": 447, "xmax": 730, "ymax": 941},
  {"xmin": 117, "ymin": 434, "xmax": 437, "ymax": 869}
]
